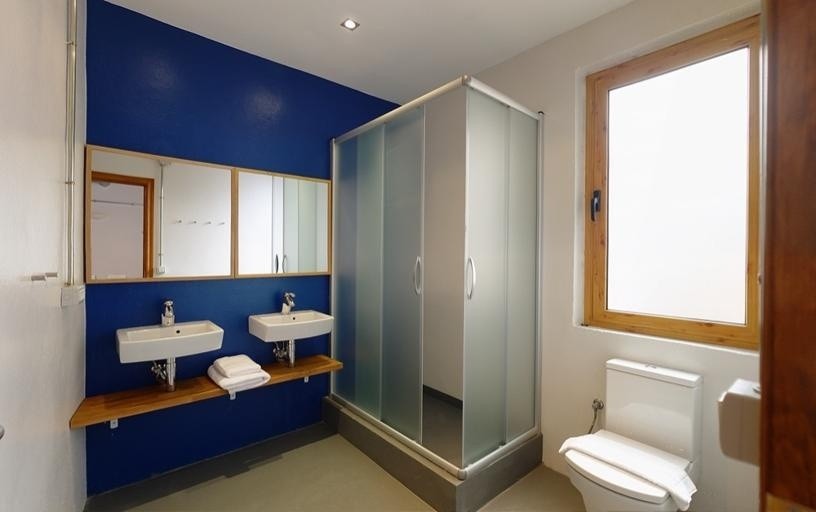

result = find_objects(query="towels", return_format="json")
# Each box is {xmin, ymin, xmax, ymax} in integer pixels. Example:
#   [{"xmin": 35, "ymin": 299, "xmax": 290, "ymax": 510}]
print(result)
[
  {"xmin": 559, "ymin": 434, "xmax": 697, "ymax": 511},
  {"xmin": 208, "ymin": 354, "xmax": 271, "ymax": 395}
]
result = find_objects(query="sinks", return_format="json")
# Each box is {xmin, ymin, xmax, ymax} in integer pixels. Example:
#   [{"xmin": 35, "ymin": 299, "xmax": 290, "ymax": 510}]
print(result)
[
  {"xmin": 115, "ymin": 320, "xmax": 225, "ymax": 363},
  {"xmin": 247, "ymin": 310, "xmax": 335, "ymax": 343}
]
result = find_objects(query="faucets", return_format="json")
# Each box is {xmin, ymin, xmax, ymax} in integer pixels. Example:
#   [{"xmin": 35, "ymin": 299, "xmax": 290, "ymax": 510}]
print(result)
[
  {"xmin": 161, "ymin": 300, "xmax": 175, "ymax": 326},
  {"xmin": 282, "ymin": 292, "xmax": 296, "ymax": 312}
]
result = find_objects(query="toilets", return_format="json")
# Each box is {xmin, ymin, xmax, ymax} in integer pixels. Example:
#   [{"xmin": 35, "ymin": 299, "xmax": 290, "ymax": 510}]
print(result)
[{"xmin": 566, "ymin": 358, "xmax": 702, "ymax": 512}]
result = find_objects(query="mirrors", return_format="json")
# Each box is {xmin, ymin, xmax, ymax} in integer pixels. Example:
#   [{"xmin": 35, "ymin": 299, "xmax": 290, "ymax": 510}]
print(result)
[{"xmin": 85, "ymin": 143, "xmax": 332, "ymax": 284}]
